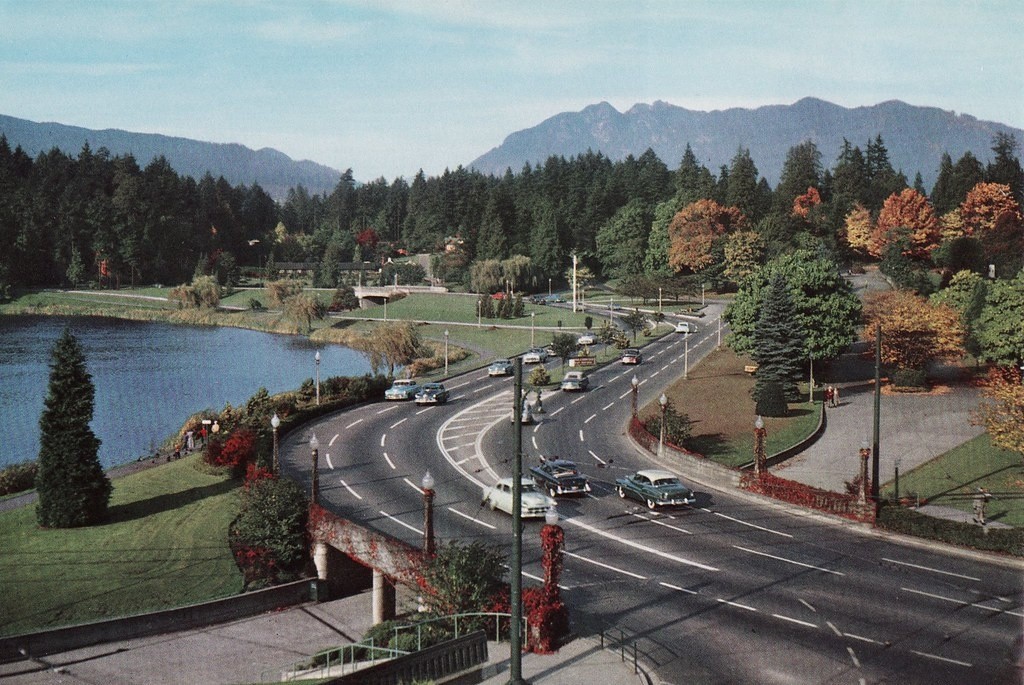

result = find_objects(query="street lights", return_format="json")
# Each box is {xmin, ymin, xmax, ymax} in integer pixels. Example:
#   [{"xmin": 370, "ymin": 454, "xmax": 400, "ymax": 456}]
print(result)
[
  {"xmin": 270, "ymin": 414, "xmax": 280, "ymax": 475},
  {"xmin": 859, "ymin": 434, "xmax": 872, "ymax": 505},
  {"xmin": 308, "ymin": 431, "xmax": 320, "ymax": 503},
  {"xmin": 542, "ymin": 502, "xmax": 563, "ymax": 594},
  {"xmin": 311, "ymin": 253, "xmax": 723, "ymax": 440},
  {"xmin": 422, "ymin": 469, "xmax": 436, "ymax": 556},
  {"xmin": 753, "ymin": 414, "xmax": 766, "ymax": 475}
]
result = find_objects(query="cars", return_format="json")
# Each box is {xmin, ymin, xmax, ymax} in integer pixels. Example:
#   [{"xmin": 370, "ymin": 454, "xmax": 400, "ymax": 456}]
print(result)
[
  {"xmin": 414, "ymin": 382, "xmax": 451, "ymax": 407},
  {"xmin": 621, "ymin": 349, "xmax": 643, "ymax": 366},
  {"xmin": 676, "ymin": 321, "xmax": 691, "ymax": 333},
  {"xmin": 489, "ymin": 359, "xmax": 514, "ymax": 377},
  {"xmin": 614, "ymin": 469, "xmax": 696, "ymax": 510},
  {"xmin": 482, "ymin": 478, "xmax": 557, "ymax": 521},
  {"xmin": 385, "ymin": 379, "xmax": 421, "ymax": 401},
  {"xmin": 523, "ymin": 347, "xmax": 549, "ymax": 365},
  {"xmin": 510, "ymin": 403, "xmax": 533, "ymax": 425},
  {"xmin": 529, "ymin": 459, "xmax": 591, "ymax": 497},
  {"xmin": 578, "ymin": 331, "xmax": 599, "ymax": 346},
  {"xmin": 560, "ymin": 370, "xmax": 590, "ymax": 392}
]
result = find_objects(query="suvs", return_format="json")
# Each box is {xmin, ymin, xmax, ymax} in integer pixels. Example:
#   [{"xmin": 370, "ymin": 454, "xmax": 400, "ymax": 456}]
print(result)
[{"xmin": 530, "ymin": 294, "xmax": 621, "ymax": 313}]
